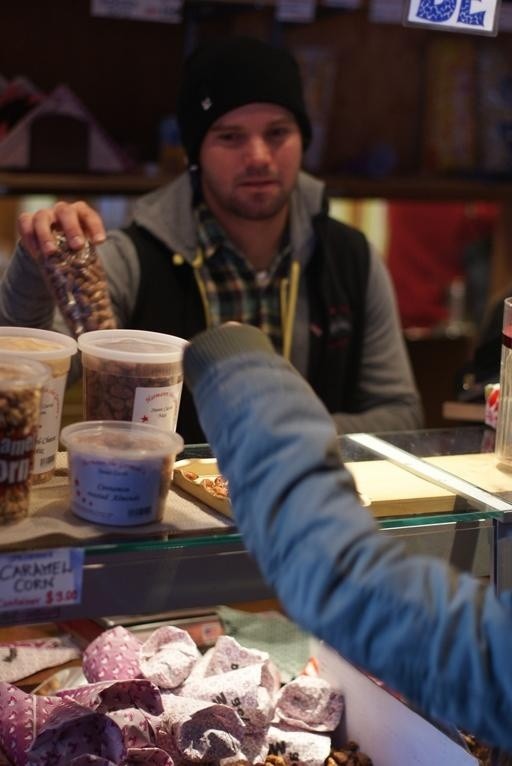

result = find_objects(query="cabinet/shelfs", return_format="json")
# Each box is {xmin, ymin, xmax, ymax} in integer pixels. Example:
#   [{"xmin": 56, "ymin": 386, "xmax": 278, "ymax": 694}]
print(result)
[
  {"xmin": 0, "ymin": 431, "xmax": 498, "ymax": 624},
  {"xmin": 347, "ymin": 421, "xmax": 512, "ymax": 598}
]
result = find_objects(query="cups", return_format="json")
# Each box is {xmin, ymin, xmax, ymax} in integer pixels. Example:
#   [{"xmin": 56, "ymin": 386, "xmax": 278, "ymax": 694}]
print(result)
[
  {"xmin": 0, "ymin": 327, "xmax": 77, "ymax": 485},
  {"xmin": 1, "ymin": 353, "xmax": 52, "ymax": 525},
  {"xmin": 80, "ymin": 328, "xmax": 188, "ymax": 437},
  {"xmin": 58, "ymin": 418, "xmax": 189, "ymax": 527},
  {"xmin": 25, "ymin": 224, "xmax": 115, "ymax": 338}
]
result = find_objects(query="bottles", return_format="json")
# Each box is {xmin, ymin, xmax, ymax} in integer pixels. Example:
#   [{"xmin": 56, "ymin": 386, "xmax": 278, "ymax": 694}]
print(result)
[{"xmin": 494, "ymin": 296, "xmax": 511, "ymax": 477}]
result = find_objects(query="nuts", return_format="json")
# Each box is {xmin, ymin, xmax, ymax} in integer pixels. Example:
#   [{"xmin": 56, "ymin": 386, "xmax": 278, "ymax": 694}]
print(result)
[
  {"xmin": 230, "ymin": 740, "xmax": 377, "ymax": 766},
  {"xmin": 62, "ymin": 418, "xmax": 183, "ymax": 526},
  {"xmin": 1, "ymin": 351, "xmax": 49, "ymax": 528},
  {"xmin": 79, "ymin": 328, "xmax": 184, "ymax": 443},
  {"xmin": 29, "ymin": 225, "xmax": 116, "ymax": 340},
  {"xmin": 1, "ymin": 324, "xmax": 78, "ymax": 486}
]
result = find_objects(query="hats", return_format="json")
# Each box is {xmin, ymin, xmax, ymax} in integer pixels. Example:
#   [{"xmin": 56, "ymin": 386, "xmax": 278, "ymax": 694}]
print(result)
[{"xmin": 174, "ymin": 34, "xmax": 314, "ymax": 172}]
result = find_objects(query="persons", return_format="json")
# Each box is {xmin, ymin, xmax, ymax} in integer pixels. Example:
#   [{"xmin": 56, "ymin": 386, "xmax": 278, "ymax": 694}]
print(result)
[
  {"xmin": 180, "ymin": 313, "xmax": 512, "ymax": 751},
  {"xmin": 0, "ymin": 36, "xmax": 426, "ymax": 446}
]
